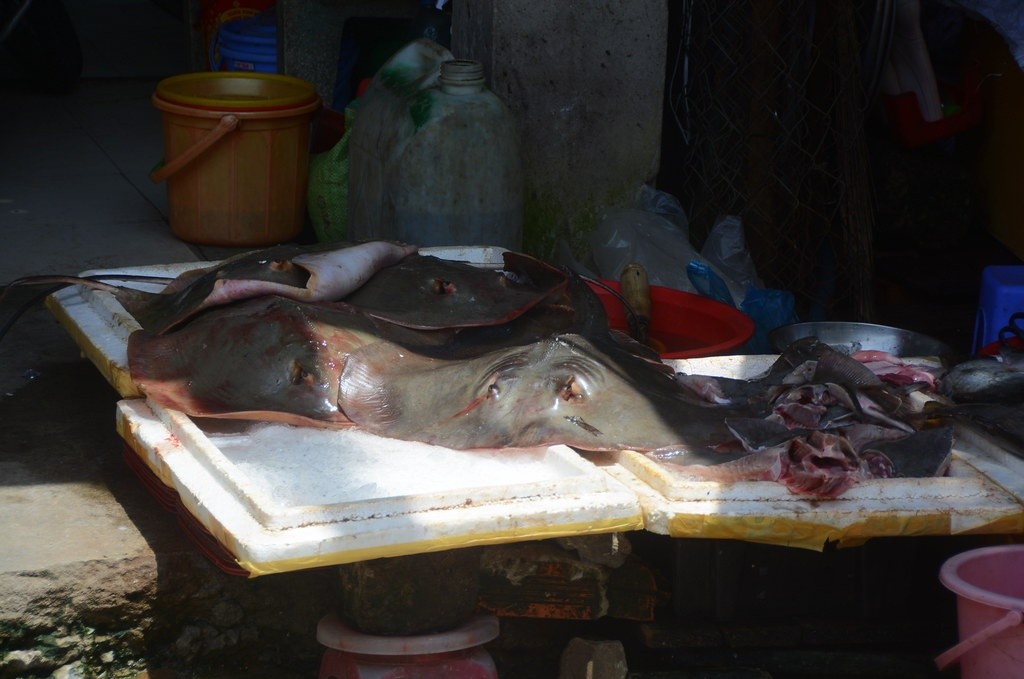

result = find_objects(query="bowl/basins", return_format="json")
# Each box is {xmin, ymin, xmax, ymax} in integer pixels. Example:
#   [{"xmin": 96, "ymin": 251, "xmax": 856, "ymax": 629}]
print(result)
[
  {"xmin": 765, "ymin": 320, "xmax": 970, "ymax": 366},
  {"xmin": 977, "ymin": 336, "xmax": 1024, "ymax": 356},
  {"xmin": 584, "ymin": 277, "xmax": 756, "ymax": 360}
]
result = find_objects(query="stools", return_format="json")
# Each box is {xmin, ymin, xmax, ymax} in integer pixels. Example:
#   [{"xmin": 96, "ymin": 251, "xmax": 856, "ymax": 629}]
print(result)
[{"xmin": 970, "ymin": 265, "xmax": 1024, "ymax": 356}]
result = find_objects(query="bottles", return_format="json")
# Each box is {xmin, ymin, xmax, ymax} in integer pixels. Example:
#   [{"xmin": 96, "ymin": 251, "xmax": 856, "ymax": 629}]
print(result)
[{"xmin": 348, "ymin": 57, "xmax": 522, "ymax": 252}]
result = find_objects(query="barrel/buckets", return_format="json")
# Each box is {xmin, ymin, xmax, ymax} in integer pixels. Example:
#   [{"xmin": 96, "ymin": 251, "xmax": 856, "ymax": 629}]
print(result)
[
  {"xmin": 313, "ymin": 99, "xmax": 344, "ymax": 153},
  {"xmin": 208, "ymin": 19, "xmax": 283, "ymax": 77},
  {"xmin": 936, "ymin": 545, "xmax": 1024, "ymax": 679},
  {"xmin": 149, "ymin": 73, "xmax": 321, "ymax": 245}
]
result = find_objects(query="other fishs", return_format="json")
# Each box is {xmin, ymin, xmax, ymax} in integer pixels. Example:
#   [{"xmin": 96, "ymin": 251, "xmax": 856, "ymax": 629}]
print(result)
[{"xmin": 7, "ymin": 239, "xmax": 815, "ymax": 451}]
